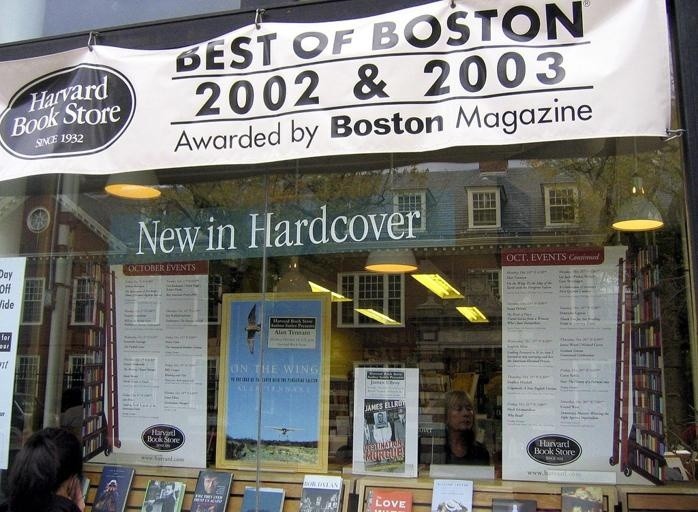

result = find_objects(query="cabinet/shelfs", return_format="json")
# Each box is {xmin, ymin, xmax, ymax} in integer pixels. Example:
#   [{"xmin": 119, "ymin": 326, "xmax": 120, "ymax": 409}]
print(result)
[
  {"xmin": 619, "ymin": 221, "xmax": 693, "ymax": 486},
  {"xmin": 67, "ymin": 255, "xmax": 111, "ymax": 463},
  {"xmin": 327, "ymin": 340, "xmax": 503, "ymax": 471}
]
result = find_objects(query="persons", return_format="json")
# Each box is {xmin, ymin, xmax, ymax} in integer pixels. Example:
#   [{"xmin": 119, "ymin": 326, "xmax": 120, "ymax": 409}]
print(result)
[
  {"xmin": 93, "ymin": 479, "xmax": 119, "ymax": 510},
  {"xmin": 202, "ymin": 473, "xmax": 221, "ymax": 494},
  {"xmin": 147, "ymin": 483, "xmax": 176, "ymax": 511},
  {"xmin": 1, "ymin": 425, "xmax": 85, "ymax": 512}
]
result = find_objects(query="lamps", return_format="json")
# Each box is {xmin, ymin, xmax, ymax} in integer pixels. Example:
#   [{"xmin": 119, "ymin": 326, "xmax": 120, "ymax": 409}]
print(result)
[
  {"xmin": 410, "ymin": 241, "xmax": 466, "ymax": 302},
  {"xmin": 270, "ymin": 157, "xmax": 313, "ymax": 295},
  {"xmin": 349, "ymin": 287, "xmax": 401, "ymax": 326},
  {"xmin": 102, "ymin": 169, "xmax": 162, "ymax": 202},
  {"xmin": 301, "ymin": 273, "xmax": 354, "ymax": 303},
  {"xmin": 362, "ymin": 155, "xmax": 417, "ymax": 274},
  {"xmin": 453, "ymin": 257, "xmax": 490, "ymax": 324},
  {"xmin": 612, "ymin": 136, "xmax": 665, "ymax": 232}
]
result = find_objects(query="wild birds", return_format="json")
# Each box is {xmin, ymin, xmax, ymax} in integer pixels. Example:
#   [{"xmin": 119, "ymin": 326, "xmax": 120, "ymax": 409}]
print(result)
[{"xmin": 244, "ymin": 304, "xmax": 261, "ymax": 353}]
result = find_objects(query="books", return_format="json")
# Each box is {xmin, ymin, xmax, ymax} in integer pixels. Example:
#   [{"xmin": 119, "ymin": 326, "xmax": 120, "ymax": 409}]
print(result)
[
  {"xmin": 428, "ymin": 478, "xmax": 474, "ymax": 512},
  {"xmin": 90, "ymin": 466, "xmax": 135, "ymax": 511},
  {"xmin": 189, "ymin": 470, "xmax": 235, "ymax": 512},
  {"xmin": 299, "ymin": 475, "xmax": 343, "ymax": 512},
  {"xmin": 366, "ymin": 487, "xmax": 413, "ymax": 512},
  {"xmin": 561, "ymin": 482, "xmax": 603, "ymax": 511},
  {"xmin": 327, "ymin": 348, "xmax": 502, "ymax": 476},
  {"xmin": 141, "ymin": 479, "xmax": 186, "ymax": 512},
  {"xmin": 491, "ymin": 496, "xmax": 536, "ymax": 512},
  {"xmin": 630, "ymin": 246, "xmax": 667, "ymax": 482},
  {"xmin": 241, "ymin": 486, "xmax": 285, "ymax": 512},
  {"xmin": 81, "ymin": 258, "xmax": 108, "ymax": 457}
]
